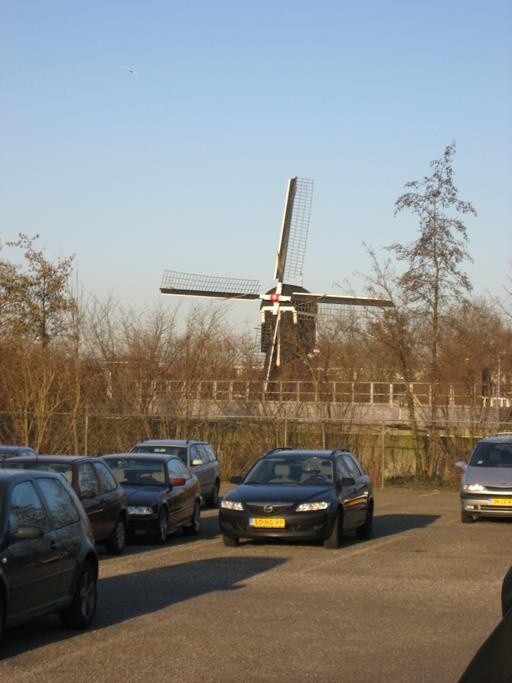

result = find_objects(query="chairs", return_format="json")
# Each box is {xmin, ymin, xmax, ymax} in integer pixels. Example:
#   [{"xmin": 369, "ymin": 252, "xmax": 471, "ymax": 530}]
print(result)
[
  {"xmin": 315, "ymin": 462, "xmax": 333, "ymax": 483},
  {"xmin": 269, "ymin": 465, "xmax": 294, "ymax": 482}
]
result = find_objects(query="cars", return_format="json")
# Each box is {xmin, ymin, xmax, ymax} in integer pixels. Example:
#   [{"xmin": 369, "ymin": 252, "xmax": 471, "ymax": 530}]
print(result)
[
  {"xmin": 0, "ymin": 467, "xmax": 98, "ymax": 630},
  {"xmin": 454, "ymin": 435, "xmax": 512, "ymax": 523},
  {"xmin": 0, "ymin": 439, "xmax": 222, "ymax": 555},
  {"xmin": 218, "ymin": 448, "xmax": 375, "ymax": 551}
]
